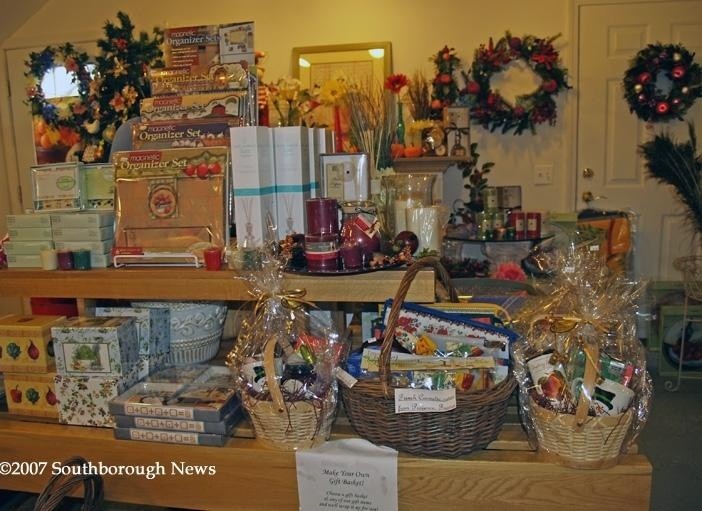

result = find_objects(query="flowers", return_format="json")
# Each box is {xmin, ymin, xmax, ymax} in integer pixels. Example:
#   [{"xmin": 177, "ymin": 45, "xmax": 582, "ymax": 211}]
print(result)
[
  {"xmin": 428, "ymin": 31, "xmax": 575, "ymax": 138},
  {"xmin": 254, "ymin": 49, "xmax": 409, "ymax": 123},
  {"xmin": 20, "ymin": 6, "xmax": 166, "ymax": 155}
]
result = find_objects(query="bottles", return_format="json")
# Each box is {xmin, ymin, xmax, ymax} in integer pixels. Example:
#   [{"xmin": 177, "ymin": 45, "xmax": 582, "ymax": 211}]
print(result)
[
  {"xmin": 475, "ymin": 185, "xmax": 540, "ymax": 238},
  {"xmin": 303, "ymin": 197, "xmax": 378, "ymax": 272}
]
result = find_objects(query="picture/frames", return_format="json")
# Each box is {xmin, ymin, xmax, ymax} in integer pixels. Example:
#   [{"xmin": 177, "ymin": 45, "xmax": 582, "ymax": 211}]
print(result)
[{"xmin": 293, "ymin": 42, "xmax": 393, "ymax": 129}]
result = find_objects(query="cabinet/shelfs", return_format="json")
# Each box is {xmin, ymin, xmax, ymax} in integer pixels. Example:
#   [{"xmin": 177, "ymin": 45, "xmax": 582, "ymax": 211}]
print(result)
[{"xmin": 0, "ymin": 251, "xmax": 654, "ymax": 511}]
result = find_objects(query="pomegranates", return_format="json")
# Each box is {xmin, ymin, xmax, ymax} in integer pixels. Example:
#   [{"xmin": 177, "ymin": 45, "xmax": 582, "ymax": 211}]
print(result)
[{"xmin": 395, "ymin": 231, "xmax": 418, "ymax": 254}]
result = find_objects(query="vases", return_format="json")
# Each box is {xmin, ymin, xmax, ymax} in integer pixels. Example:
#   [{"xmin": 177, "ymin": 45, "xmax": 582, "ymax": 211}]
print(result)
[{"xmin": 396, "ymin": 103, "xmax": 406, "ymax": 145}]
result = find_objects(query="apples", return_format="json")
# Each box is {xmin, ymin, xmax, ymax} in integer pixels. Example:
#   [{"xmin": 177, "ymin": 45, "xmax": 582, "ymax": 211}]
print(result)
[
  {"xmin": 10, "ymin": 385, "xmax": 22, "ymax": 402},
  {"xmin": 184, "ymin": 162, "xmax": 221, "ymax": 178}
]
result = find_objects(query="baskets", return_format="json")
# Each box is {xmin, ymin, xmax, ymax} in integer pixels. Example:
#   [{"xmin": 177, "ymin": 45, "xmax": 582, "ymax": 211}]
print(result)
[
  {"xmin": 241, "ymin": 335, "xmax": 339, "ymax": 452},
  {"xmin": 529, "ymin": 343, "xmax": 636, "ymax": 471},
  {"xmin": 340, "ymin": 256, "xmax": 518, "ymax": 460}
]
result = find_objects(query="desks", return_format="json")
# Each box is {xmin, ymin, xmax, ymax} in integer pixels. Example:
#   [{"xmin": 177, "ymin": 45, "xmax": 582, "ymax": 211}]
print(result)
[{"xmin": 444, "ymin": 225, "xmax": 555, "ymax": 276}]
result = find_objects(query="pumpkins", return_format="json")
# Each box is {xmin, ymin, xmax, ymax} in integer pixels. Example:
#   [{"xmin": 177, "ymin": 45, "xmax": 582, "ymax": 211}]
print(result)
[{"xmin": 390, "ymin": 140, "xmax": 421, "ymax": 158}]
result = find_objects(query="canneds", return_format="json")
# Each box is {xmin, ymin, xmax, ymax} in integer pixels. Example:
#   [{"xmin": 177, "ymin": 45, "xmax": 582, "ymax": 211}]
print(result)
[
  {"xmin": 478, "ymin": 186, "xmax": 504, "ymax": 239},
  {"xmin": 527, "ymin": 213, "xmax": 542, "ymax": 237},
  {"xmin": 512, "ymin": 211, "xmax": 525, "ymax": 236}
]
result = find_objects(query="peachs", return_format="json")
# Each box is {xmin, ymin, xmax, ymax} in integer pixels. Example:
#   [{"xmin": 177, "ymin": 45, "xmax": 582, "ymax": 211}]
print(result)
[{"xmin": 541, "ymin": 372, "xmax": 563, "ymax": 398}]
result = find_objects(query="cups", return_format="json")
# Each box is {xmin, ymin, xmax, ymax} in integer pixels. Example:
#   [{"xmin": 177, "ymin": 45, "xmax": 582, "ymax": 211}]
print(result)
[
  {"xmin": 39, "ymin": 245, "xmax": 92, "ymax": 272},
  {"xmin": 570, "ymin": 377, "xmax": 635, "ymax": 416},
  {"xmin": 203, "ymin": 248, "xmax": 222, "ymax": 272}
]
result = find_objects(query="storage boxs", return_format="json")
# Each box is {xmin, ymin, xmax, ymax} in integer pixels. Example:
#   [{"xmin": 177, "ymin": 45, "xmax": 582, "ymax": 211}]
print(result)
[{"xmin": 0, "ymin": 307, "xmax": 173, "ymax": 427}]
